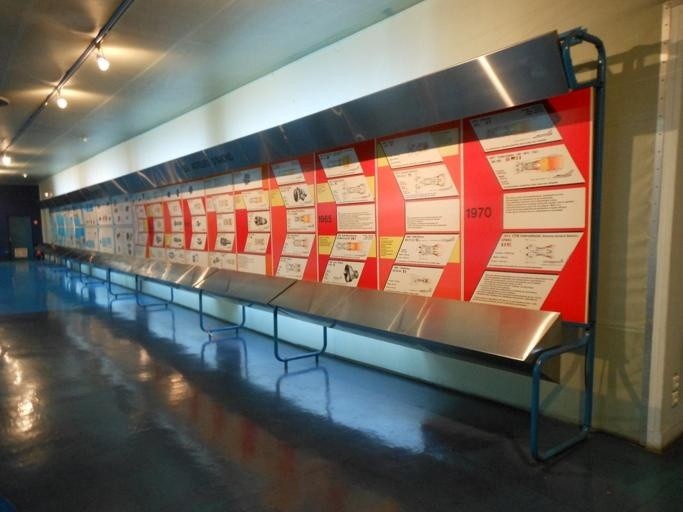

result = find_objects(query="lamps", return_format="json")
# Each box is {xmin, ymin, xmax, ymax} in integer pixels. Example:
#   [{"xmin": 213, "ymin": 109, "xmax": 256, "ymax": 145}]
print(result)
[
  {"xmin": 95, "ymin": 44, "xmax": 110, "ymax": 72},
  {"xmin": 57, "ymin": 87, "xmax": 68, "ymax": 109}
]
